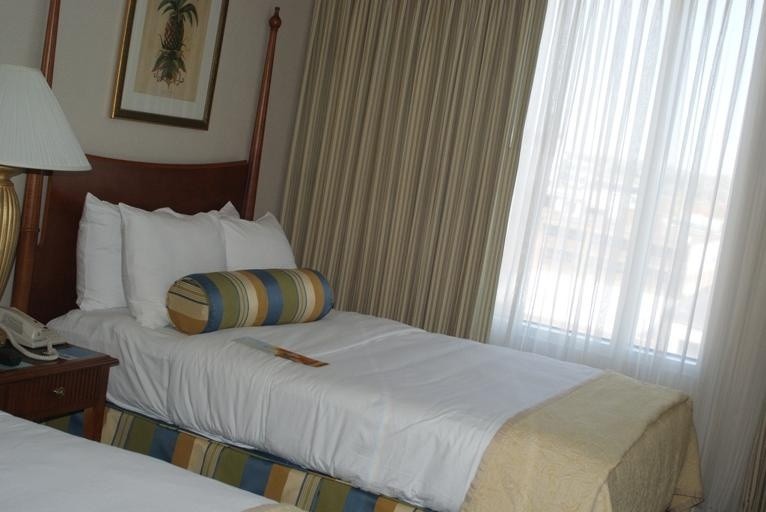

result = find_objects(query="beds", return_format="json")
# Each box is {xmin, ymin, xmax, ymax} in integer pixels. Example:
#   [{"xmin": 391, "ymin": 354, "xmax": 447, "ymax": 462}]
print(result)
[
  {"xmin": 10, "ymin": 0, "xmax": 705, "ymax": 512},
  {"xmin": 1, "ymin": 410, "xmax": 310, "ymax": 512}
]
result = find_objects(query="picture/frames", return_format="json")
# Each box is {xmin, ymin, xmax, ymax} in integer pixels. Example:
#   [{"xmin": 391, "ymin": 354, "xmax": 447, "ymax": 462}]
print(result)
[{"xmin": 109, "ymin": 0, "xmax": 229, "ymax": 130}]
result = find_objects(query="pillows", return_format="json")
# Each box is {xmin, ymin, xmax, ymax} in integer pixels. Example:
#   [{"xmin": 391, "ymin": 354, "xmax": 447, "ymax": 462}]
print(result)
[{"xmin": 75, "ymin": 192, "xmax": 335, "ymax": 334}]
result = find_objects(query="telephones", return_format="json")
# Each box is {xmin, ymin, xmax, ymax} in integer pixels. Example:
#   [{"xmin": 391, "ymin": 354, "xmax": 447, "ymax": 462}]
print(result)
[{"xmin": 0, "ymin": 306, "xmax": 66, "ymax": 348}]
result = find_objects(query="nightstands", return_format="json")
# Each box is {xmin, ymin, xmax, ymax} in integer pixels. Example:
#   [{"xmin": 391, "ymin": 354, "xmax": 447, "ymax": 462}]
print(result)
[{"xmin": 1, "ymin": 343, "xmax": 120, "ymax": 442}]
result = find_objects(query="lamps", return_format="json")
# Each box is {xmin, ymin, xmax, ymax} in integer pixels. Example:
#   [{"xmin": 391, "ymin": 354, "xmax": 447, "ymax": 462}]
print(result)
[{"xmin": 0, "ymin": 63, "xmax": 92, "ymax": 347}]
